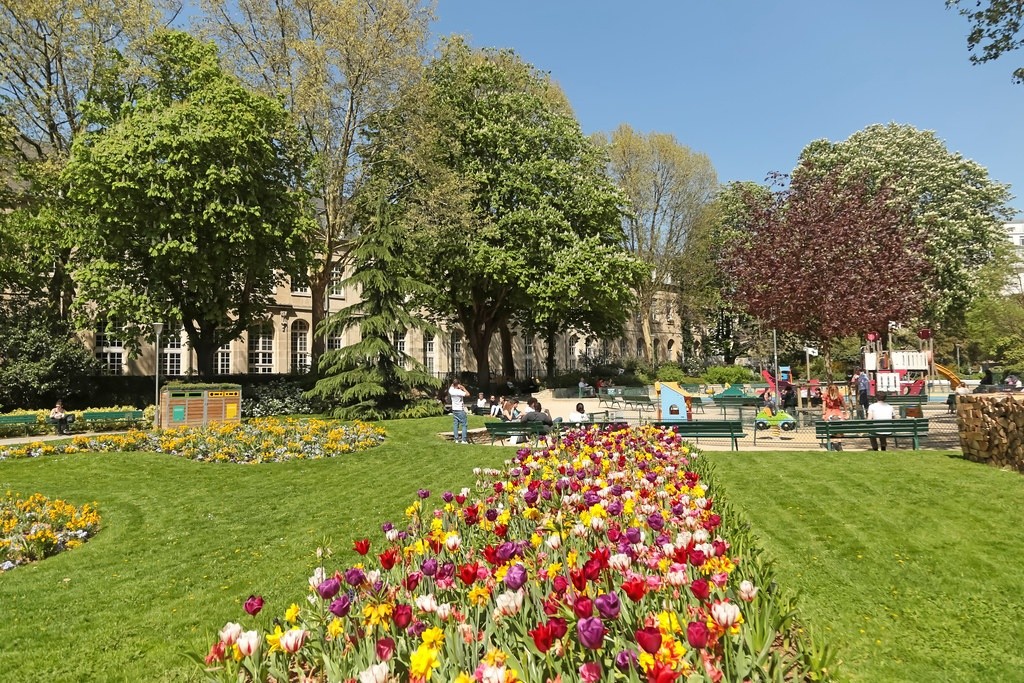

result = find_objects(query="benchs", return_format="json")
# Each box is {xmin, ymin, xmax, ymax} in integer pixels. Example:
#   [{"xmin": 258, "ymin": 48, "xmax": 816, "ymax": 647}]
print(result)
[
  {"xmin": 792, "ymin": 396, "xmax": 822, "ymax": 407},
  {"xmin": 554, "ymin": 421, "xmax": 627, "ymax": 431},
  {"xmin": 484, "ymin": 421, "xmax": 549, "ymax": 447},
  {"xmin": 83, "ymin": 411, "xmax": 147, "ymax": 432},
  {"xmin": 467, "ymin": 406, "xmax": 503, "ymax": 416},
  {"xmin": 0, "ymin": 414, "xmax": 40, "ymax": 437},
  {"xmin": 814, "ymin": 418, "xmax": 929, "ymax": 451},
  {"xmin": 45, "ymin": 414, "xmax": 78, "ymax": 434},
  {"xmin": 682, "ymin": 383, "xmax": 707, "ymax": 413},
  {"xmin": 714, "ymin": 397, "xmax": 765, "ymax": 417},
  {"xmin": 577, "ymin": 385, "xmax": 657, "ymax": 412},
  {"xmin": 651, "ymin": 420, "xmax": 749, "ymax": 451},
  {"xmin": 870, "ymin": 396, "xmax": 928, "ymax": 418}
]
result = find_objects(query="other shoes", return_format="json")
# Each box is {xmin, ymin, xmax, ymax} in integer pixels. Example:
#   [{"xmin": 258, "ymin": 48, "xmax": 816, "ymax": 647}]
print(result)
[
  {"xmin": 461, "ymin": 441, "xmax": 468, "ymax": 444},
  {"xmin": 455, "ymin": 440, "xmax": 458, "ymax": 444}
]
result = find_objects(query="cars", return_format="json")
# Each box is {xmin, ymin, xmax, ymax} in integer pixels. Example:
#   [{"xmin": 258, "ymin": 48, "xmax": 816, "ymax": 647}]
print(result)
[{"xmin": 754, "ymin": 406, "xmax": 797, "ymax": 432}]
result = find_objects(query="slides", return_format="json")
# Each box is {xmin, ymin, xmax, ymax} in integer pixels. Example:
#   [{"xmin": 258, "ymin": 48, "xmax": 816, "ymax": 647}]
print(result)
[{"xmin": 934, "ymin": 362, "xmax": 962, "ymax": 389}]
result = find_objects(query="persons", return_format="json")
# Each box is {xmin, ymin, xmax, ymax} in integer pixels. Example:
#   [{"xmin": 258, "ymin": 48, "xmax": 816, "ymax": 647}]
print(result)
[
  {"xmin": 578, "ymin": 377, "xmax": 596, "ymax": 397},
  {"xmin": 821, "ymin": 384, "xmax": 850, "ymax": 452},
  {"xmin": 946, "ymin": 382, "xmax": 970, "ymax": 413},
  {"xmin": 568, "ymin": 402, "xmax": 590, "ymax": 423},
  {"xmin": 476, "ymin": 392, "xmax": 563, "ymax": 434},
  {"xmin": 810, "ymin": 388, "xmax": 823, "ymax": 403},
  {"xmin": 780, "ymin": 386, "xmax": 799, "ymax": 418},
  {"xmin": 51, "ymin": 398, "xmax": 71, "ymax": 435},
  {"xmin": 597, "ymin": 378, "xmax": 615, "ymax": 388},
  {"xmin": 864, "ymin": 390, "xmax": 898, "ymax": 451},
  {"xmin": 760, "ymin": 387, "xmax": 776, "ymax": 417},
  {"xmin": 853, "ymin": 366, "xmax": 871, "ymax": 418},
  {"xmin": 449, "ymin": 379, "xmax": 471, "ymax": 445}
]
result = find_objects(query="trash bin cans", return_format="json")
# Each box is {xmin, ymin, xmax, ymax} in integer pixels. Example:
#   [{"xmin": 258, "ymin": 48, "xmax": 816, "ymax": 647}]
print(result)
[{"xmin": 160, "ymin": 384, "xmax": 243, "ymax": 430}]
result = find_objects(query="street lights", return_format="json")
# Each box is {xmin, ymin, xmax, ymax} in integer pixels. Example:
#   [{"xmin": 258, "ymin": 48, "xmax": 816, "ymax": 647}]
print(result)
[
  {"xmin": 955, "ymin": 343, "xmax": 962, "ymax": 375},
  {"xmin": 152, "ymin": 322, "xmax": 163, "ymax": 429}
]
